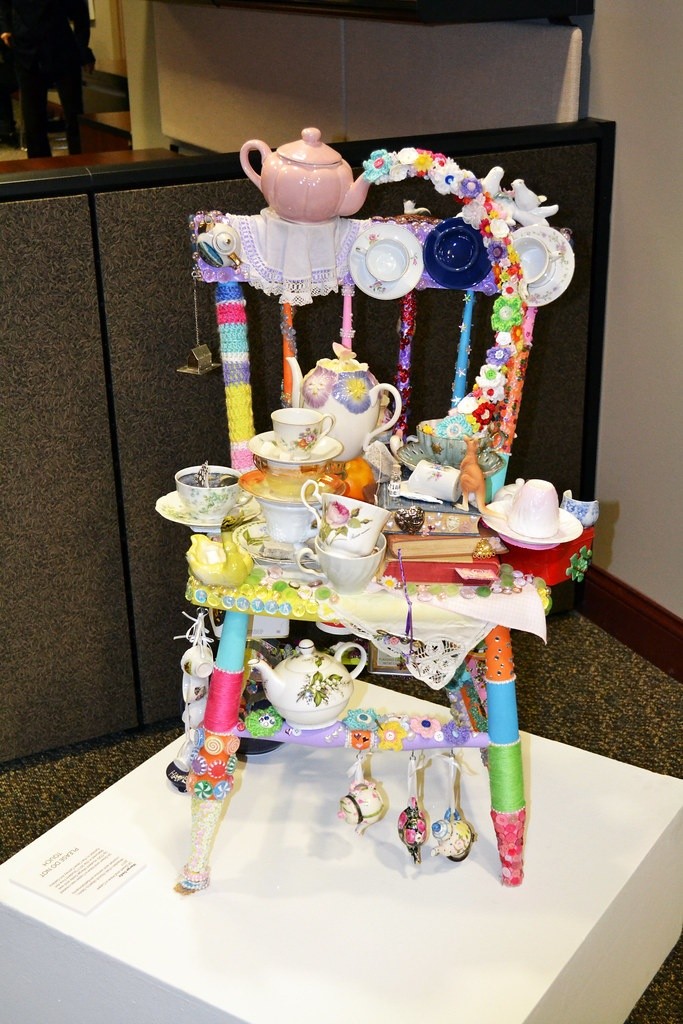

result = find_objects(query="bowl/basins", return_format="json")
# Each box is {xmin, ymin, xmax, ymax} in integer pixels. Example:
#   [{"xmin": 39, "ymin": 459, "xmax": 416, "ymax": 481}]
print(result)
[
  {"xmin": 416, "ymin": 419, "xmax": 507, "ymax": 469},
  {"xmin": 250, "ymin": 454, "xmax": 333, "ymax": 498}
]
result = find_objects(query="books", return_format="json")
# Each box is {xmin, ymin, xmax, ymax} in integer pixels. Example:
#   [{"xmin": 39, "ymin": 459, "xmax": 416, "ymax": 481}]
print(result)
[{"xmin": 362, "ymin": 464, "xmax": 510, "ymax": 588}]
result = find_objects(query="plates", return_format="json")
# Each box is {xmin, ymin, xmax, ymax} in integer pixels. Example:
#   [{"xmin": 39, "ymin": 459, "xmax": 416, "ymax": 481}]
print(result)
[
  {"xmin": 154, "ymin": 490, "xmax": 261, "ymax": 532},
  {"xmin": 246, "ymin": 429, "xmax": 345, "ymax": 469},
  {"xmin": 505, "ymin": 222, "xmax": 576, "ymax": 309},
  {"xmin": 422, "ymin": 217, "xmax": 495, "ymax": 291},
  {"xmin": 482, "ymin": 501, "xmax": 583, "ymax": 549},
  {"xmin": 232, "ymin": 518, "xmax": 317, "ymax": 568},
  {"xmin": 346, "ymin": 222, "xmax": 424, "ymax": 301},
  {"xmin": 238, "ymin": 469, "xmax": 347, "ymax": 506},
  {"xmin": 396, "ymin": 443, "xmax": 505, "ymax": 481}
]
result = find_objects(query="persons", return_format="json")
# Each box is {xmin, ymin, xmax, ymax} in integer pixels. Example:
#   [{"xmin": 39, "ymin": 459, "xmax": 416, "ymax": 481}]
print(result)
[
  {"xmin": 0, "ymin": 28, "xmax": 66, "ymax": 148},
  {"xmin": 0, "ymin": 0, "xmax": 95, "ymax": 162}
]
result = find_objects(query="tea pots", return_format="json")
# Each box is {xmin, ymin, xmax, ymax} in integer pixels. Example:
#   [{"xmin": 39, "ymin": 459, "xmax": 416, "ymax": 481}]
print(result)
[
  {"xmin": 237, "ymin": 127, "xmax": 376, "ymax": 226},
  {"xmin": 248, "ymin": 640, "xmax": 366, "ymax": 732},
  {"xmin": 397, "ymin": 796, "xmax": 429, "ymax": 865},
  {"xmin": 430, "ymin": 809, "xmax": 473, "ymax": 861},
  {"xmin": 337, "ymin": 780, "xmax": 386, "ymax": 837},
  {"xmin": 195, "ymin": 220, "xmax": 245, "ymax": 270}
]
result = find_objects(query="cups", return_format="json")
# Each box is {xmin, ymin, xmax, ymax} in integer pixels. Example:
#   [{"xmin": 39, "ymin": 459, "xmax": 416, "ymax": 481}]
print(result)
[
  {"xmin": 316, "ymin": 621, "xmax": 353, "ymax": 635},
  {"xmin": 174, "ymin": 465, "xmax": 243, "ymax": 520},
  {"xmin": 560, "ymin": 490, "xmax": 599, "ymax": 530},
  {"xmin": 269, "ymin": 407, "xmax": 335, "ymax": 461},
  {"xmin": 494, "ymin": 477, "xmax": 525, "ymax": 501},
  {"xmin": 356, "ymin": 240, "xmax": 411, "ymax": 284},
  {"xmin": 172, "ymin": 645, "xmax": 215, "ymax": 774},
  {"xmin": 510, "ymin": 237, "xmax": 562, "ymax": 287},
  {"xmin": 431, "ymin": 225, "xmax": 480, "ymax": 275},
  {"xmin": 407, "ymin": 457, "xmax": 463, "ymax": 505},
  {"xmin": 253, "ymin": 496, "xmax": 325, "ymax": 545},
  {"xmin": 299, "ymin": 479, "xmax": 395, "ymax": 596},
  {"xmin": 504, "ymin": 478, "xmax": 564, "ymax": 538}
]
